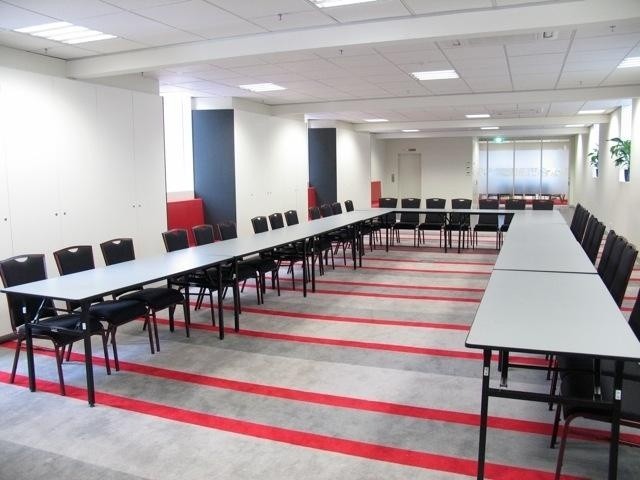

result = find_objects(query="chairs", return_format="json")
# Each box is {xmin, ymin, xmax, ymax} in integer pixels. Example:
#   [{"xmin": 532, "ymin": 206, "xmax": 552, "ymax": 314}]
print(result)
[
  {"xmin": 473, "ymin": 198, "xmax": 499, "ymax": 250},
  {"xmin": 0, "ymin": 253, "xmax": 111, "ymax": 396},
  {"xmin": 546, "ymin": 204, "xmax": 640, "ymax": 480},
  {"xmin": 499, "ymin": 198, "xmax": 526, "ymax": 251},
  {"xmin": 162, "ymin": 231, "xmax": 239, "ymax": 326},
  {"xmin": 442, "ymin": 198, "xmax": 472, "ymax": 249},
  {"xmin": 53, "ymin": 245, "xmax": 152, "ymax": 371},
  {"xmin": 309, "ymin": 208, "xmax": 354, "ymax": 269},
  {"xmin": 417, "ymin": 198, "xmax": 449, "ymax": 248},
  {"xmin": 374, "ymin": 198, "xmax": 398, "ymax": 248},
  {"xmin": 284, "ymin": 210, "xmax": 334, "ymax": 273},
  {"xmin": 321, "ymin": 200, "xmax": 375, "ymax": 259},
  {"xmin": 269, "ymin": 214, "xmax": 323, "ymax": 282},
  {"xmin": 532, "ymin": 200, "xmax": 554, "ymax": 210},
  {"xmin": 191, "ymin": 224, "xmax": 259, "ymax": 314},
  {"xmin": 392, "ymin": 197, "xmax": 421, "ymax": 248},
  {"xmin": 102, "ymin": 237, "xmax": 190, "ymax": 352},
  {"xmin": 216, "ymin": 222, "xmax": 281, "ymax": 304},
  {"xmin": 250, "ymin": 216, "xmax": 308, "ymax": 289}
]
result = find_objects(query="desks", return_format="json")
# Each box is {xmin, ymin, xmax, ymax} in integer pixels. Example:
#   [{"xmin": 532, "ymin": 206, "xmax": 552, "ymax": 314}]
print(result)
[
  {"xmin": 371, "ymin": 180, "xmax": 382, "ymax": 202},
  {"xmin": 334, "ymin": 210, "xmax": 391, "ymax": 267},
  {"xmin": 169, "ymin": 230, "xmax": 313, "ymax": 333},
  {"xmin": 0, "ymin": 250, "xmax": 234, "ymax": 408},
  {"xmin": 307, "ymin": 188, "xmax": 318, "ymax": 216},
  {"xmin": 462, "ymin": 213, "xmax": 638, "ymax": 478},
  {"xmin": 372, "ymin": 208, "xmax": 557, "ymax": 243},
  {"xmin": 272, "ymin": 219, "xmax": 361, "ymax": 291}
]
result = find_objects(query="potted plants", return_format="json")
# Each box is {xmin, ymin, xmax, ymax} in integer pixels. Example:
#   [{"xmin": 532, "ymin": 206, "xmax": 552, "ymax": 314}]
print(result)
[
  {"xmin": 589, "ymin": 144, "xmax": 599, "ymax": 178},
  {"xmin": 606, "ymin": 134, "xmax": 630, "ymax": 183}
]
